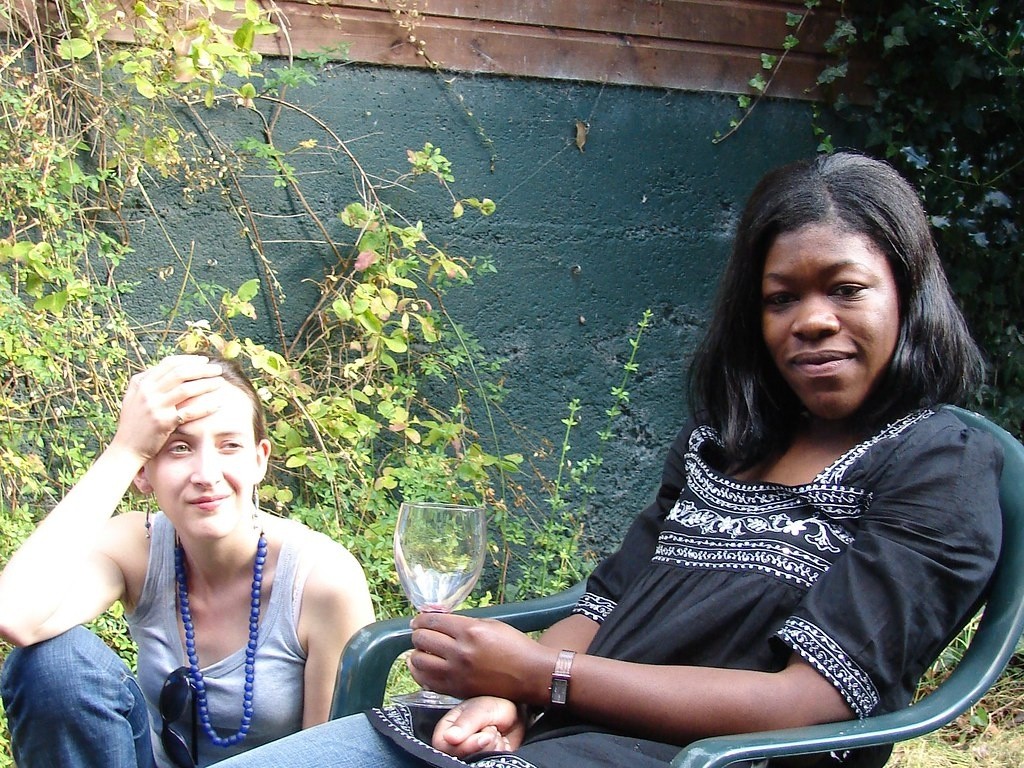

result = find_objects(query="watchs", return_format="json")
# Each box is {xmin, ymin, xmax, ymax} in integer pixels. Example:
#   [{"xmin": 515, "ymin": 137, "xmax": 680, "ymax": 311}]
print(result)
[{"xmin": 552, "ymin": 650, "xmax": 577, "ymax": 704}]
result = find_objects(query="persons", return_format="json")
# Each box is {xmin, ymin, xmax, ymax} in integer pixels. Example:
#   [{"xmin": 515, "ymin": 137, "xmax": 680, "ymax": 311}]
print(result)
[
  {"xmin": 0, "ymin": 352, "xmax": 375, "ymax": 768},
  {"xmin": 203, "ymin": 152, "xmax": 1001, "ymax": 768}
]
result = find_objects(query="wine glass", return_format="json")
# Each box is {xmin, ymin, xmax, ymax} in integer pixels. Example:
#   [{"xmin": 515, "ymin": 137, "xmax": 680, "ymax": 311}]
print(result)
[{"xmin": 390, "ymin": 501, "xmax": 487, "ymax": 708}]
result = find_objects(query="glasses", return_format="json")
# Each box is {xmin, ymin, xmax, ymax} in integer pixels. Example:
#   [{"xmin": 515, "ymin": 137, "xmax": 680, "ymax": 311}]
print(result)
[{"xmin": 159, "ymin": 666, "xmax": 198, "ymax": 768}]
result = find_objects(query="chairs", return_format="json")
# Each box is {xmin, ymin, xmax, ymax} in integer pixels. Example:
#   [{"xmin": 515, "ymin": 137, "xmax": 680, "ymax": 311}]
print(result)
[{"xmin": 326, "ymin": 404, "xmax": 1024, "ymax": 768}]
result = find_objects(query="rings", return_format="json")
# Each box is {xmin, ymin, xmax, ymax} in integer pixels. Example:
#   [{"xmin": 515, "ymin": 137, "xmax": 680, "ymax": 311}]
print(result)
[{"xmin": 178, "ymin": 411, "xmax": 184, "ymax": 425}]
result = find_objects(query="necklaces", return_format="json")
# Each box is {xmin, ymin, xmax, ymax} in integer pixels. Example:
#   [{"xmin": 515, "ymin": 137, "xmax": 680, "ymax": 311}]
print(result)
[{"xmin": 175, "ymin": 528, "xmax": 267, "ymax": 746}]
